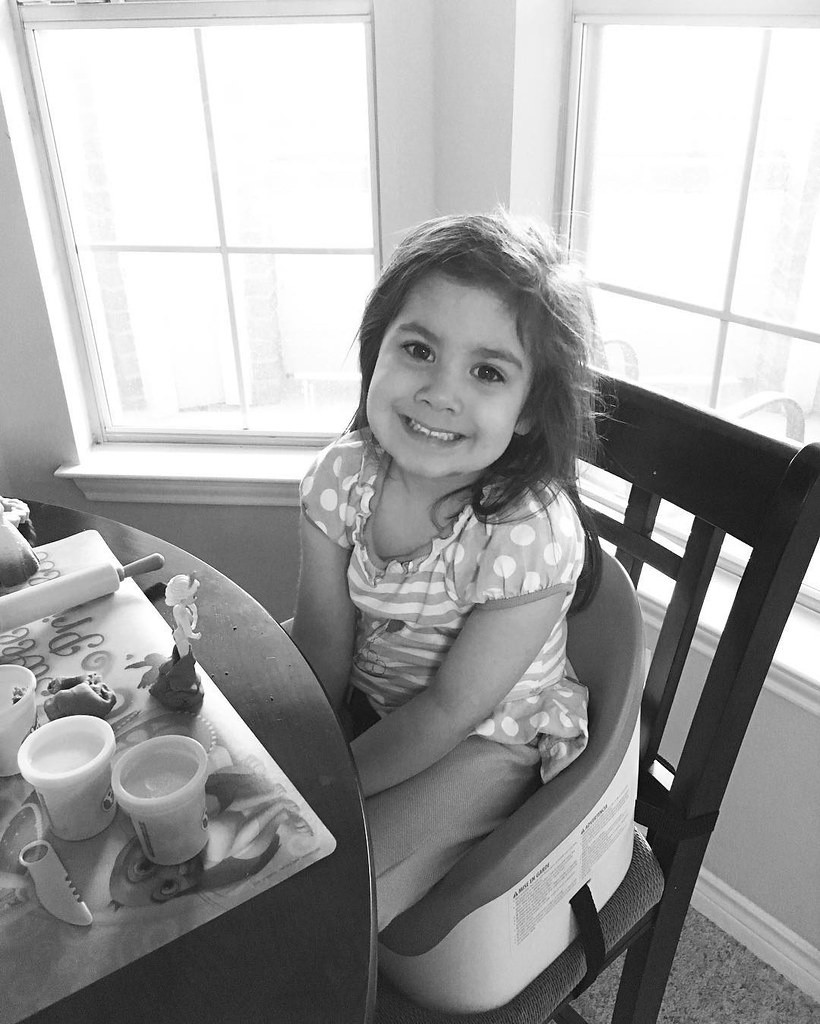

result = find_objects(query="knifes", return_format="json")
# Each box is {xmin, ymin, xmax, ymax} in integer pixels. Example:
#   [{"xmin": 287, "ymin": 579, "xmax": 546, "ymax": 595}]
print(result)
[{"xmin": 19, "ymin": 840, "xmax": 92, "ymax": 926}]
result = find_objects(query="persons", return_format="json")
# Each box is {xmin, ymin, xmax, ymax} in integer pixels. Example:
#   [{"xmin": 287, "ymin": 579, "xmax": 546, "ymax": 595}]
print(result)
[{"xmin": 287, "ymin": 211, "xmax": 602, "ymax": 934}]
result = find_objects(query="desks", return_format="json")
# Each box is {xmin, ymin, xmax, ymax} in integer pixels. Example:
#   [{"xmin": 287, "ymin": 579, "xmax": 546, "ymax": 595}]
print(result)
[{"xmin": 0, "ymin": 496, "xmax": 380, "ymax": 1024}]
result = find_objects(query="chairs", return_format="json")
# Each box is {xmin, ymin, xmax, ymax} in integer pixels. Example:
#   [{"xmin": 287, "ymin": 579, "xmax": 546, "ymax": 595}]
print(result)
[{"xmin": 282, "ymin": 356, "xmax": 820, "ymax": 1024}]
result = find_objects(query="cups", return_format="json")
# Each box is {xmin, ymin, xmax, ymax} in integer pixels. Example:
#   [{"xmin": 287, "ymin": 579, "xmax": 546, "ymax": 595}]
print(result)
[
  {"xmin": 111, "ymin": 735, "xmax": 209, "ymax": 864},
  {"xmin": 0, "ymin": 665, "xmax": 40, "ymax": 776},
  {"xmin": 18, "ymin": 715, "xmax": 116, "ymax": 841}
]
